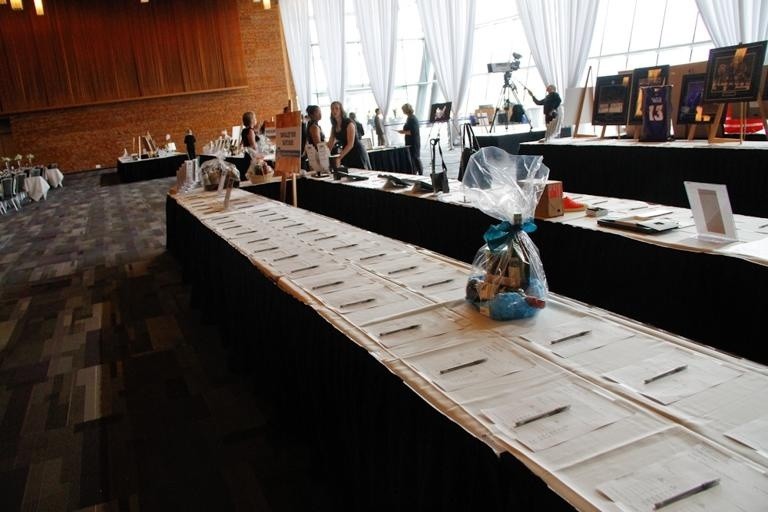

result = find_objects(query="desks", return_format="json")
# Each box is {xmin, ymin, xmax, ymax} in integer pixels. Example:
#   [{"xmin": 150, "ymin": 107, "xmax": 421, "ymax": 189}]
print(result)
[
  {"xmin": 519, "ymin": 139, "xmax": 768, "ymax": 214},
  {"xmin": 200, "ymin": 146, "xmax": 413, "ymax": 172},
  {"xmin": 167, "ymin": 169, "xmax": 768, "ymax": 512},
  {"xmin": 118, "ymin": 152, "xmax": 188, "ymax": 182},
  {"xmin": 464, "ymin": 123, "xmax": 571, "ymax": 152}
]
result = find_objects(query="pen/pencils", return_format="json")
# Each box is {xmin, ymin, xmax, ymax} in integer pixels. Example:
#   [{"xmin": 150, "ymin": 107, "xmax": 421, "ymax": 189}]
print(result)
[
  {"xmin": 423, "ymin": 278, "xmax": 453, "ymax": 288},
  {"xmin": 652, "ymin": 477, "xmax": 720, "ymax": 510},
  {"xmin": 233, "ymin": 201, "xmax": 247, "ymax": 205},
  {"xmin": 273, "ymin": 254, "xmax": 298, "ymax": 261},
  {"xmin": 552, "ymin": 330, "xmax": 590, "ymax": 344},
  {"xmin": 269, "ymin": 217, "xmax": 288, "ymax": 222},
  {"xmin": 197, "ymin": 207, "xmax": 213, "ymax": 210},
  {"xmin": 189, "ymin": 197, "xmax": 201, "ymax": 200},
  {"xmin": 255, "ymin": 247, "xmax": 279, "ymax": 253},
  {"xmin": 360, "ymin": 253, "xmax": 386, "ymax": 260},
  {"xmin": 333, "ymin": 243, "xmax": 358, "ymax": 250},
  {"xmin": 759, "ymin": 223, "xmax": 768, "ymax": 229},
  {"xmin": 223, "ymin": 225, "xmax": 242, "ymax": 230},
  {"xmin": 591, "ymin": 200, "xmax": 608, "ymax": 205},
  {"xmin": 192, "ymin": 204, "xmax": 207, "ymax": 207},
  {"xmin": 236, "ymin": 231, "xmax": 257, "ymax": 235},
  {"xmin": 572, "ymin": 196, "xmax": 582, "ymax": 200},
  {"xmin": 284, "ymin": 223, "xmax": 304, "ymax": 228},
  {"xmin": 630, "ymin": 206, "xmax": 648, "ymax": 211},
  {"xmin": 516, "ymin": 404, "xmax": 571, "ymax": 427},
  {"xmin": 297, "ymin": 229, "xmax": 319, "ymax": 235},
  {"xmin": 252, "ymin": 209, "xmax": 269, "ymax": 214},
  {"xmin": 237, "ymin": 205, "xmax": 254, "ymax": 209},
  {"xmin": 259, "ymin": 212, "xmax": 277, "ymax": 217},
  {"xmin": 190, "ymin": 201, "xmax": 204, "ymax": 204},
  {"xmin": 203, "ymin": 210, "xmax": 220, "ymax": 214},
  {"xmin": 340, "ymin": 298, "xmax": 375, "ymax": 308},
  {"xmin": 229, "ymin": 198, "xmax": 240, "ymax": 201},
  {"xmin": 185, "ymin": 195, "xmax": 197, "ymax": 198},
  {"xmin": 388, "ymin": 266, "xmax": 417, "ymax": 274},
  {"xmin": 216, "ymin": 220, "xmax": 235, "ymax": 224},
  {"xmin": 440, "ymin": 357, "xmax": 487, "ymax": 374},
  {"xmin": 380, "ymin": 324, "xmax": 420, "ymax": 336},
  {"xmin": 313, "ymin": 281, "xmax": 344, "ymax": 290},
  {"xmin": 212, "ymin": 216, "xmax": 229, "ymax": 220},
  {"xmin": 315, "ymin": 235, "xmax": 336, "ymax": 241},
  {"xmin": 291, "ymin": 265, "xmax": 319, "ymax": 273},
  {"xmin": 248, "ymin": 238, "xmax": 269, "ymax": 243},
  {"xmin": 644, "ymin": 364, "xmax": 687, "ymax": 384}
]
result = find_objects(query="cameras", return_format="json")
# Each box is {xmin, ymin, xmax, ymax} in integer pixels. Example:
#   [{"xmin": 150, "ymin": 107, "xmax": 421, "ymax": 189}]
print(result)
[{"xmin": 487, "ymin": 52, "xmax": 522, "ymax": 73}]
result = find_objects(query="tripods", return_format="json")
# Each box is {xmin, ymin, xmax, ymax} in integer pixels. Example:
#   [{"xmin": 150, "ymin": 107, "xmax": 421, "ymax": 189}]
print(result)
[{"xmin": 489, "ymin": 84, "xmax": 533, "ymax": 133}]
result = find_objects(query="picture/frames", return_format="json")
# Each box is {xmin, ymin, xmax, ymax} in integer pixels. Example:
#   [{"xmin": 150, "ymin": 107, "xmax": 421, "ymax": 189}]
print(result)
[
  {"xmin": 703, "ymin": 40, "xmax": 767, "ymax": 104},
  {"xmin": 430, "ymin": 102, "xmax": 451, "ymax": 121},
  {"xmin": 591, "ymin": 74, "xmax": 628, "ymax": 126},
  {"xmin": 678, "ymin": 75, "xmax": 714, "ymax": 124},
  {"xmin": 629, "ymin": 65, "xmax": 670, "ymax": 123}
]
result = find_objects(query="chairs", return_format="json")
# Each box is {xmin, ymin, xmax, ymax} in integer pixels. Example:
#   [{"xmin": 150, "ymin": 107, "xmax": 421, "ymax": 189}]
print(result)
[
  {"xmin": 0, "ymin": 178, "xmax": 18, "ymax": 215},
  {"xmin": 13, "ymin": 174, "xmax": 26, "ymax": 208}
]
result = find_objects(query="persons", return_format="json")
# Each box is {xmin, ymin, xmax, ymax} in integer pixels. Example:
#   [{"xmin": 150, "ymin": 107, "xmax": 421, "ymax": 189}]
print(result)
[
  {"xmin": 284, "ymin": 106, "xmax": 288, "ymax": 113},
  {"xmin": 327, "ymin": 101, "xmax": 372, "ymax": 170},
  {"xmin": 241, "ymin": 129, "xmax": 277, "ymax": 182},
  {"xmin": 260, "ymin": 120, "xmax": 268, "ymax": 135},
  {"xmin": 526, "ymin": 84, "xmax": 562, "ymax": 138},
  {"xmin": 240, "ymin": 112, "xmax": 261, "ymax": 181},
  {"xmin": 398, "ymin": 104, "xmax": 423, "ymax": 175},
  {"xmin": 301, "ymin": 114, "xmax": 306, "ymax": 156},
  {"xmin": 184, "ymin": 128, "xmax": 197, "ymax": 160},
  {"xmin": 349, "ymin": 113, "xmax": 364, "ymax": 142},
  {"xmin": 375, "ymin": 108, "xmax": 386, "ymax": 146},
  {"xmin": 306, "ymin": 105, "xmax": 325, "ymax": 152}
]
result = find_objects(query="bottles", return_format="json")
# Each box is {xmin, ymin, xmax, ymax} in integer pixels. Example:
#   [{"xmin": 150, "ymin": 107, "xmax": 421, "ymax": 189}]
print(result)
[
  {"xmin": 465, "ymin": 279, "xmax": 548, "ymax": 308},
  {"xmin": 508, "ymin": 213, "xmax": 531, "ymax": 292},
  {"xmin": 200, "ymin": 168, "xmax": 212, "ymax": 190}
]
